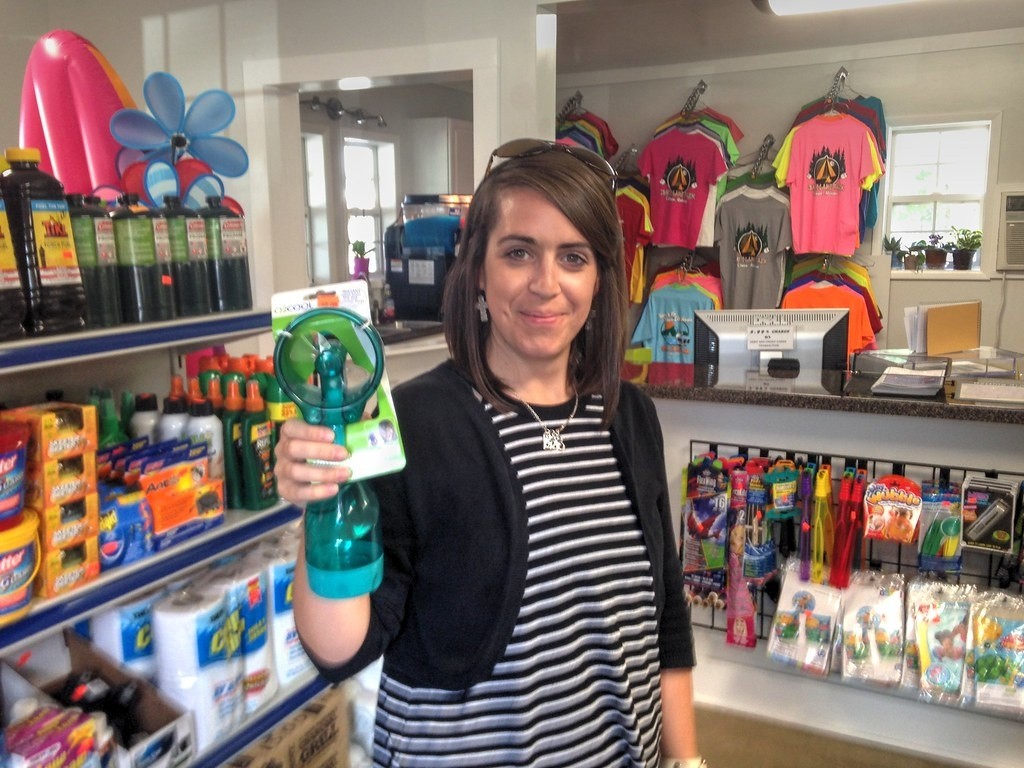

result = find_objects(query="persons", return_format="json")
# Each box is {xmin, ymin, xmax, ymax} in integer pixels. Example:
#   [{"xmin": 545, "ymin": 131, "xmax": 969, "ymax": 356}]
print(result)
[{"xmin": 273, "ymin": 145, "xmax": 707, "ymax": 768}]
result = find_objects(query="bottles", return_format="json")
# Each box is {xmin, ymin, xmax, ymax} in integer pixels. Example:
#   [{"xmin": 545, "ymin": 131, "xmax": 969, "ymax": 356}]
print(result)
[
  {"xmin": 65, "ymin": 191, "xmax": 255, "ymax": 329},
  {"xmin": 379, "ymin": 284, "xmax": 395, "ymax": 324},
  {"xmin": 86, "ymin": 383, "xmax": 226, "ymax": 495},
  {"xmin": 0, "ymin": 146, "xmax": 88, "ymax": 343},
  {"xmin": 163, "ymin": 352, "xmax": 298, "ymax": 511}
]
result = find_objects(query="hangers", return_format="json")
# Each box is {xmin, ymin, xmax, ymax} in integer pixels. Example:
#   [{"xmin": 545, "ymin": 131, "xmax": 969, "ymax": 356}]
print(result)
[
  {"xmin": 609, "ymin": 145, "xmax": 636, "ymax": 187},
  {"xmin": 816, "ymin": 252, "xmax": 845, "ymax": 288},
  {"xmin": 736, "ymin": 138, "xmax": 779, "ymax": 196},
  {"xmin": 820, "ymin": 72, "xmax": 857, "ymax": 117},
  {"xmin": 673, "ymin": 251, "xmax": 707, "ymax": 287},
  {"xmin": 676, "ymin": 85, "xmax": 708, "ymax": 135},
  {"xmin": 555, "ymin": 93, "xmax": 587, "ymax": 139}
]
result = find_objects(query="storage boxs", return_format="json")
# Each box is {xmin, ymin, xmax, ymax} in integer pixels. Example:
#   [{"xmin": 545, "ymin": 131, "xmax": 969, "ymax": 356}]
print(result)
[
  {"xmin": 0, "ymin": 401, "xmax": 224, "ymax": 600},
  {"xmin": 212, "ymin": 682, "xmax": 355, "ymax": 768},
  {"xmin": 0, "ymin": 626, "xmax": 198, "ymax": 768}
]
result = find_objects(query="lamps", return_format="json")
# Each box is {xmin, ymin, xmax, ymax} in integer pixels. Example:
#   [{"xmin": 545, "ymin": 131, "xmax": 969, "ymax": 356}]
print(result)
[{"xmin": 298, "ymin": 95, "xmax": 387, "ymax": 128}]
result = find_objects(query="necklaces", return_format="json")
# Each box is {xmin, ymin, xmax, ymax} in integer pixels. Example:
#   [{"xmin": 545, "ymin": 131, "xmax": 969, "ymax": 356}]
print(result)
[{"xmin": 513, "ymin": 384, "xmax": 579, "ymax": 452}]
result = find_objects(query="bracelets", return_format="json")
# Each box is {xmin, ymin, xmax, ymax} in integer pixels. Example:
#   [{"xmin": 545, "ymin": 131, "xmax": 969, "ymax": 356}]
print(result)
[{"xmin": 665, "ymin": 757, "xmax": 705, "ymax": 768}]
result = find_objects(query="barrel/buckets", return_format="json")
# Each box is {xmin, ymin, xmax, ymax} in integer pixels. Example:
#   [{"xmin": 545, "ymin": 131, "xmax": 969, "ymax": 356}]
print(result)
[
  {"xmin": 0, "ymin": 506, "xmax": 41, "ymax": 614},
  {"xmin": 0, "ymin": 421, "xmax": 32, "ymax": 533}
]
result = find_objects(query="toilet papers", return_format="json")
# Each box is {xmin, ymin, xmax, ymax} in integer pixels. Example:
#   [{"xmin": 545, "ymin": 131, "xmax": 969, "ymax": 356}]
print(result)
[{"xmin": 90, "ymin": 523, "xmax": 317, "ymax": 752}]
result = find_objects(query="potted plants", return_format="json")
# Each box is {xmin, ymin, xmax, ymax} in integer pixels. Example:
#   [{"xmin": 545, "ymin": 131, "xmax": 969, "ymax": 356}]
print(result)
[
  {"xmin": 946, "ymin": 226, "xmax": 982, "ymax": 270},
  {"xmin": 352, "ymin": 240, "xmax": 376, "ymax": 279},
  {"xmin": 897, "ymin": 239, "xmax": 928, "ymax": 273},
  {"xmin": 883, "ymin": 234, "xmax": 902, "ymax": 269},
  {"xmin": 925, "ymin": 233, "xmax": 947, "ymax": 269}
]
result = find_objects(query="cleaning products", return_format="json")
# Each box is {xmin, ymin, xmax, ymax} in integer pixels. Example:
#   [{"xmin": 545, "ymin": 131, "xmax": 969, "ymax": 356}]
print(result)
[{"xmin": 92, "ymin": 355, "xmax": 314, "ymax": 514}]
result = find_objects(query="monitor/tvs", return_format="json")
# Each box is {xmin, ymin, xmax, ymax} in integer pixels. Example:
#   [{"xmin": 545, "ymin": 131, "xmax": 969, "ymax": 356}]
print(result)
[{"xmin": 694, "ymin": 308, "xmax": 850, "ymax": 372}]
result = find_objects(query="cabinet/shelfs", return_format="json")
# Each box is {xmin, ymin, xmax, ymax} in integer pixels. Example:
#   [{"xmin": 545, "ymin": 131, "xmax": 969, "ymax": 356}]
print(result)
[{"xmin": 0, "ymin": 309, "xmax": 334, "ymax": 768}]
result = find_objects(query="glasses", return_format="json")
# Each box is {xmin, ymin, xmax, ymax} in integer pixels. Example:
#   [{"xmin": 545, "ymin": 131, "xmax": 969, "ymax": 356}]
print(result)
[{"xmin": 486, "ymin": 139, "xmax": 619, "ymax": 208}]
